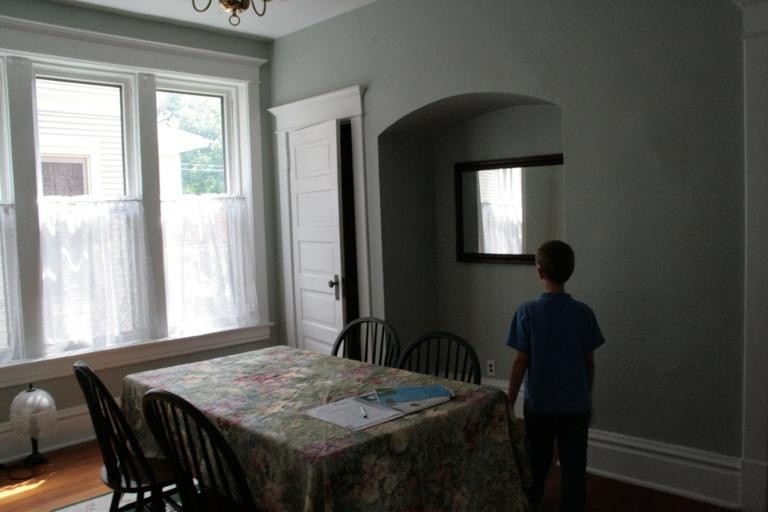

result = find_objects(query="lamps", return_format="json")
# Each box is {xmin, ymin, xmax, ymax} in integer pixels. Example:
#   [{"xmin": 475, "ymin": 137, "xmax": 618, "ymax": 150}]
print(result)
[
  {"xmin": 8, "ymin": 382, "xmax": 59, "ymax": 469},
  {"xmin": 191, "ymin": 0, "xmax": 270, "ymax": 26}
]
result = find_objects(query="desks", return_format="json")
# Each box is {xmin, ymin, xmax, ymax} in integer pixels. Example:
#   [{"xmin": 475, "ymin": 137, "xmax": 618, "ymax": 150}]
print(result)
[{"xmin": 120, "ymin": 345, "xmax": 512, "ymax": 512}]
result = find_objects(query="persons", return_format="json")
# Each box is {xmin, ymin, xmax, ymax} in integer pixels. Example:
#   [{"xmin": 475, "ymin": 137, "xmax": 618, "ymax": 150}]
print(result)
[{"xmin": 506, "ymin": 241, "xmax": 608, "ymax": 510}]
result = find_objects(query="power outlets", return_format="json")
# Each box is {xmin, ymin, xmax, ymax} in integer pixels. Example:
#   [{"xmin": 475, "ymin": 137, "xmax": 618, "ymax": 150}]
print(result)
[{"xmin": 487, "ymin": 360, "xmax": 495, "ymax": 377}]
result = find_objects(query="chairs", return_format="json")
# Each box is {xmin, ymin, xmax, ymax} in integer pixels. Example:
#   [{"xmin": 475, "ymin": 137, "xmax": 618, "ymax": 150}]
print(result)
[
  {"xmin": 396, "ymin": 332, "xmax": 481, "ymax": 385},
  {"xmin": 331, "ymin": 318, "xmax": 399, "ymax": 369},
  {"xmin": 72, "ymin": 360, "xmax": 175, "ymax": 512},
  {"xmin": 141, "ymin": 389, "xmax": 256, "ymax": 512}
]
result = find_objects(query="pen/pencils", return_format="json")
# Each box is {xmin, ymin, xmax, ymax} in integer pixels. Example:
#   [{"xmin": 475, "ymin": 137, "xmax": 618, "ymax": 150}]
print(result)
[{"xmin": 360, "ymin": 407, "xmax": 367, "ymax": 418}]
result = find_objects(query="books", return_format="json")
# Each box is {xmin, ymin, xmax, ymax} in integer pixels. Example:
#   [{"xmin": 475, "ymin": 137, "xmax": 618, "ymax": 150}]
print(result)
[
  {"xmin": 306, "ymin": 391, "xmax": 449, "ymax": 433},
  {"xmin": 356, "ymin": 392, "xmax": 382, "ymax": 409},
  {"xmin": 375, "ymin": 382, "xmax": 455, "ymax": 405}
]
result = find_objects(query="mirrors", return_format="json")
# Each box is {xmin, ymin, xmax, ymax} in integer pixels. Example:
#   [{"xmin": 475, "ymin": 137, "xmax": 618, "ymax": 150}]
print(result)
[{"xmin": 454, "ymin": 153, "xmax": 564, "ymax": 265}]
